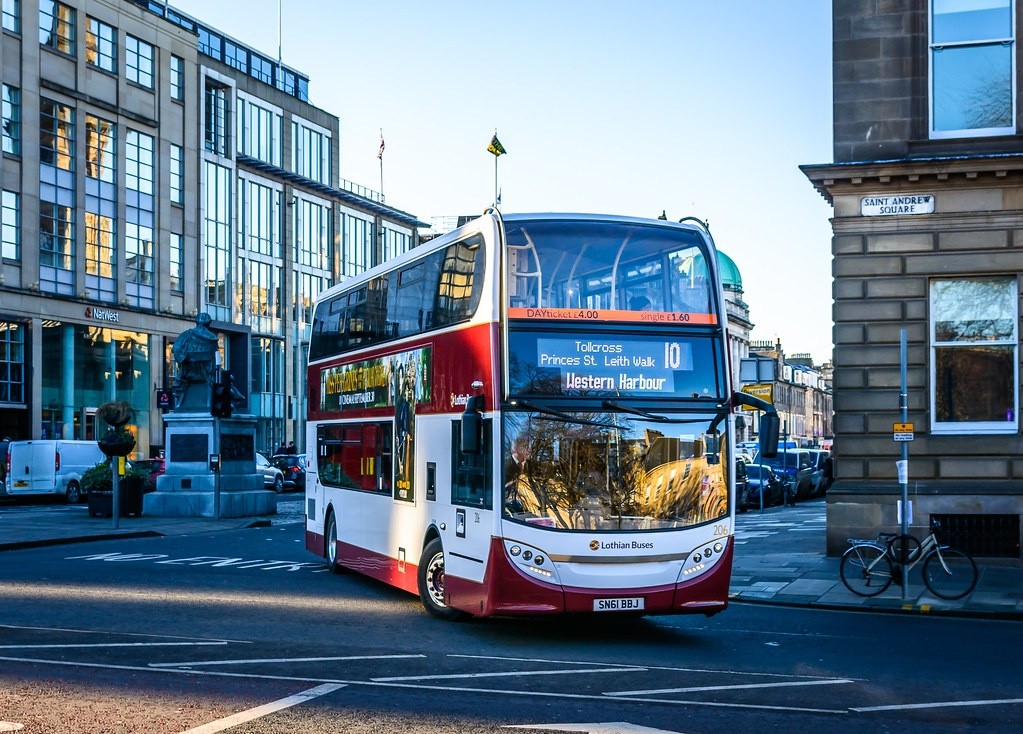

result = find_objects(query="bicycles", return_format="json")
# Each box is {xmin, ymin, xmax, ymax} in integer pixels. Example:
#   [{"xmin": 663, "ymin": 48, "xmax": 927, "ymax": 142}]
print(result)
[{"xmin": 839, "ymin": 515, "xmax": 978, "ymax": 600}]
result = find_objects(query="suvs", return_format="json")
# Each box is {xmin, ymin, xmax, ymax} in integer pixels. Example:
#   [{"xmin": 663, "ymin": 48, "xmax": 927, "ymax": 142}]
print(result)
[
  {"xmin": 808, "ymin": 450, "xmax": 835, "ymax": 500},
  {"xmin": 750, "ymin": 449, "xmax": 814, "ymax": 505}
]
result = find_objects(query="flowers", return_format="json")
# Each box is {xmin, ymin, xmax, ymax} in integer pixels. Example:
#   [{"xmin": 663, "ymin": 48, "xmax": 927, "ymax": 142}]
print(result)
[{"xmin": 101, "ymin": 429, "xmax": 135, "ymax": 443}]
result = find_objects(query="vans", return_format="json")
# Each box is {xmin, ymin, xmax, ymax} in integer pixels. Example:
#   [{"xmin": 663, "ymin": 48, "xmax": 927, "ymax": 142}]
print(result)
[{"xmin": 4, "ymin": 439, "xmax": 133, "ymax": 504}]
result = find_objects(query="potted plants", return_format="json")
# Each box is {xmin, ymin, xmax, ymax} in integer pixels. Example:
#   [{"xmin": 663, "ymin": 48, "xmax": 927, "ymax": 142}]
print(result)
[
  {"xmin": 80, "ymin": 459, "xmax": 149, "ymax": 517},
  {"xmin": 94, "ymin": 401, "xmax": 137, "ymax": 426}
]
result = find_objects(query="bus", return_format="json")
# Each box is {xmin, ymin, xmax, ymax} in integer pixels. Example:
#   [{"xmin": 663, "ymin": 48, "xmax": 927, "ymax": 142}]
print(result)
[{"xmin": 305, "ymin": 208, "xmax": 782, "ymax": 620}]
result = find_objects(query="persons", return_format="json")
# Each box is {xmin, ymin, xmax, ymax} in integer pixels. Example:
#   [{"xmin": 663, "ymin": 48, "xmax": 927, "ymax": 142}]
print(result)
[
  {"xmin": 629, "ymin": 295, "xmax": 652, "ymax": 310},
  {"xmin": 286, "ymin": 441, "xmax": 297, "ymax": 454},
  {"xmin": 0, "ymin": 435, "xmax": 12, "ymax": 484},
  {"xmin": 505, "ymin": 437, "xmax": 539, "ymax": 514},
  {"xmin": 171, "ymin": 312, "xmax": 247, "ymax": 402},
  {"xmin": 577, "ymin": 443, "xmax": 610, "ymax": 496},
  {"xmin": 276, "ymin": 442, "xmax": 288, "ymax": 455}
]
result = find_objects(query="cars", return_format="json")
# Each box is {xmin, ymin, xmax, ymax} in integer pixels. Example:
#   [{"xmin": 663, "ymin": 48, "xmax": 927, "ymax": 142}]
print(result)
[
  {"xmin": 135, "ymin": 458, "xmax": 164, "ymax": 484},
  {"xmin": 254, "ymin": 453, "xmax": 284, "ymax": 493},
  {"xmin": 269, "ymin": 454, "xmax": 307, "ymax": 493},
  {"xmin": 734, "ymin": 455, "xmax": 751, "ymax": 513},
  {"xmin": 744, "ymin": 464, "xmax": 784, "ymax": 510}
]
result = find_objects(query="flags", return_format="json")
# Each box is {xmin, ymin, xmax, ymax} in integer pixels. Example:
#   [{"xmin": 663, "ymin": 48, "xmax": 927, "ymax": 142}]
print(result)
[
  {"xmin": 487, "ymin": 135, "xmax": 507, "ymax": 158},
  {"xmin": 377, "ymin": 138, "xmax": 386, "ymax": 160}
]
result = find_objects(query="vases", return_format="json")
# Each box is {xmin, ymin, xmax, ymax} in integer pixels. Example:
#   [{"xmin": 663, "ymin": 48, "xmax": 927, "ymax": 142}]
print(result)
[{"xmin": 98, "ymin": 441, "xmax": 136, "ymax": 456}]
